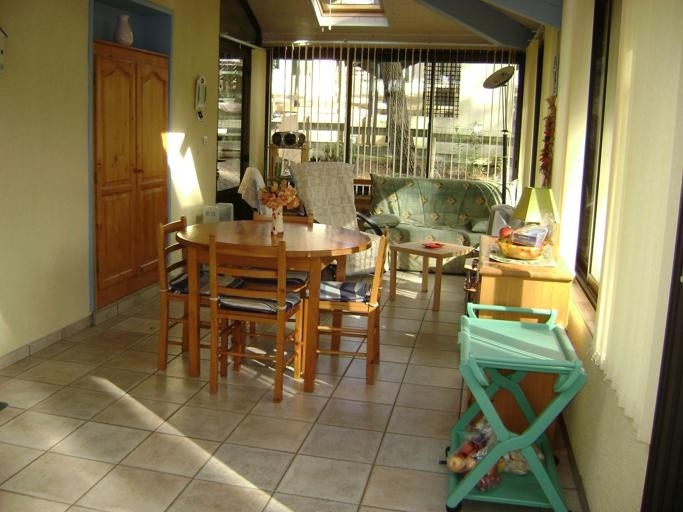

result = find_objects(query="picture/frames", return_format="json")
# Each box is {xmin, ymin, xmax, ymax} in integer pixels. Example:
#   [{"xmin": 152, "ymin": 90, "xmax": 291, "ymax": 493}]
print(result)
[{"xmin": 195, "ymin": 75, "xmax": 208, "ymax": 111}]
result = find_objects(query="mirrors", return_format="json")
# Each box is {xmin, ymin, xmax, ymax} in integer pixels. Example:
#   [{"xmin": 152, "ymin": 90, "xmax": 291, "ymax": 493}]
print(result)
[{"xmin": 216, "ymin": 50, "xmax": 251, "ymax": 199}]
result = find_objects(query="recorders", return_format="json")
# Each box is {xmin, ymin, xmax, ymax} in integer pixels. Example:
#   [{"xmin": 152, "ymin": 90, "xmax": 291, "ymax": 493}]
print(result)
[{"xmin": 272, "ymin": 132, "xmax": 306, "ymax": 148}]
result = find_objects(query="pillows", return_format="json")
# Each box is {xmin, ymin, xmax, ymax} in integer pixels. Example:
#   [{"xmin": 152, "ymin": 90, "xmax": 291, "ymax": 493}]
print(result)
[
  {"xmin": 467, "ymin": 216, "xmax": 489, "ymax": 232},
  {"xmin": 363, "ymin": 213, "xmax": 401, "ymax": 228}
]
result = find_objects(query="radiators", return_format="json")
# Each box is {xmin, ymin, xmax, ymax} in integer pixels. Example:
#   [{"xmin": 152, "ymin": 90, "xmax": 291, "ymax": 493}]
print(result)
[{"xmin": 202, "ymin": 202, "xmax": 235, "ymax": 224}]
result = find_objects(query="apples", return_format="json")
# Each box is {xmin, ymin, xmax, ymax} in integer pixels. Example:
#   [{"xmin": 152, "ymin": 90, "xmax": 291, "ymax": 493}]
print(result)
[
  {"xmin": 447, "ymin": 456, "xmax": 465, "ymax": 474},
  {"xmin": 500, "ymin": 227, "xmax": 513, "ymax": 239}
]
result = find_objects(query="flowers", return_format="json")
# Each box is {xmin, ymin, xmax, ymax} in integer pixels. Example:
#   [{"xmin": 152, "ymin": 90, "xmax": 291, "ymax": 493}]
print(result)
[
  {"xmin": 256, "ymin": 179, "xmax": 300, "ymax": 209},
  {"xmin": 538, "ymin": 94, "xmax": 557, "ymax": 188}
]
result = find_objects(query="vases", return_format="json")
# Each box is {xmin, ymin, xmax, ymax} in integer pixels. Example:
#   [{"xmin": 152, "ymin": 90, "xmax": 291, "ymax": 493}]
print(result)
[
  {"xmin": 271, "ymin": 204, "xmax": 284, "ymax": 233},
  {"xmin": 114, "ymin": 13, "xmax": 135, "ymax": 46}
]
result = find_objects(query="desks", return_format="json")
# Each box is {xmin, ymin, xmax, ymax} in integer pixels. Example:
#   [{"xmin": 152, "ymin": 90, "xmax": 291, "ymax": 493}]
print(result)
[{"xmin": 388, "ymin": 240, "xmax": 475, "ymax": 311}]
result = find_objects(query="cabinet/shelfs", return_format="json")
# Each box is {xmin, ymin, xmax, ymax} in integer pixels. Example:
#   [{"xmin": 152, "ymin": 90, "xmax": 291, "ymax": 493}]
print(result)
[
  {"xmin": 444, "ymin": 302, "xmax": 589, "ymax": 512},
  {"xmin": 268, "ymin": 144, "xmax": 310, "ymax": 193},
  {"xmin": 91, "ymin": 38, "xmax": 170, "ymax": 309},
  {"xmin": 464, "ymin": 235, "xmax": 576, "ymax": 442}
]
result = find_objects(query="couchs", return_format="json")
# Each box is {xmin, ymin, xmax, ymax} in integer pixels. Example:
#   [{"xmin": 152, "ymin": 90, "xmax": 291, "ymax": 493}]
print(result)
[{"xmin": 365, "ymin": 173, "xmax": 521, "ymax": 275}]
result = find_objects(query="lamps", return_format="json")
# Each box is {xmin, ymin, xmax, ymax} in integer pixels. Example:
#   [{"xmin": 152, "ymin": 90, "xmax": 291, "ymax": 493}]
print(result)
[
  {"xmin": 483, "ymin": 66, "xmax": 514, "ymax": 204},
  {"xmin": 514, "ymin": 185, "xmax": 559, "ymax": 235}
]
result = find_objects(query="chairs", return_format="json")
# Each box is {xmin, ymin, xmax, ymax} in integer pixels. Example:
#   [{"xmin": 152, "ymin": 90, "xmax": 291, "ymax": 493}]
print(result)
[
  {"xmin": 289, "ymin": 162, "xmax": 388, "ymax": 278},
  {"xmin": 237, "ymin": 169, "xmax": 266, "ymax": 213}
]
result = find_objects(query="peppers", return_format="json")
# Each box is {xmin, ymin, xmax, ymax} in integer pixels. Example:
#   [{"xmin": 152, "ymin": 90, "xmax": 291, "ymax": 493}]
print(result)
[{"xmin": 538, "ymin": 115, "xmax": 555, "ymax": 186}]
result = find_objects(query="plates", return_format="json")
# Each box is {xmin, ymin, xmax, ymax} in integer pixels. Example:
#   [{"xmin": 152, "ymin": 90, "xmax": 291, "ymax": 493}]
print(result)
[{"xmin": 422, "ymin": 242, "xmax": 445, "ymax": 248}]
